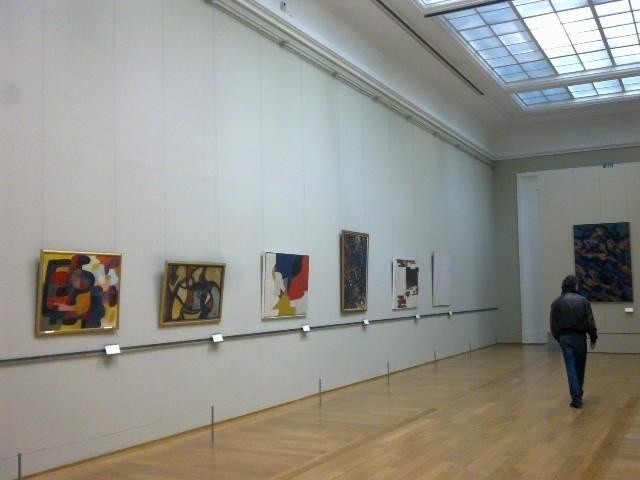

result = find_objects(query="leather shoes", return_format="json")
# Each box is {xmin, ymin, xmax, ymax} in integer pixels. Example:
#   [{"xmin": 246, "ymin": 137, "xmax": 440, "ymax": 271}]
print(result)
[{"xmin": 570, "ymin": 398, "xmax": 583, "ymax": 408}]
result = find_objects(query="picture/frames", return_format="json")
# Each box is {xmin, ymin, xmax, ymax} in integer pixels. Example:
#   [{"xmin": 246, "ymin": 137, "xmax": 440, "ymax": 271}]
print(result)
[
  {"xmin": 342, "ymin": 231, "xmax": 369, "ymax": 312},
  {"xmin": 36, "ymin": 250, "xmax": 123, "ymax": 337},
  {"xmin": 159, "ymin": 261, "xmax": 226, "ymax": 327}
]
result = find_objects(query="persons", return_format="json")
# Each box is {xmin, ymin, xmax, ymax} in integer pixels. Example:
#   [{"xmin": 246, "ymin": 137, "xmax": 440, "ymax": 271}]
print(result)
[{"xmin": 549, "ymin": 274, "xmax": 598, "ymax": 409}]
